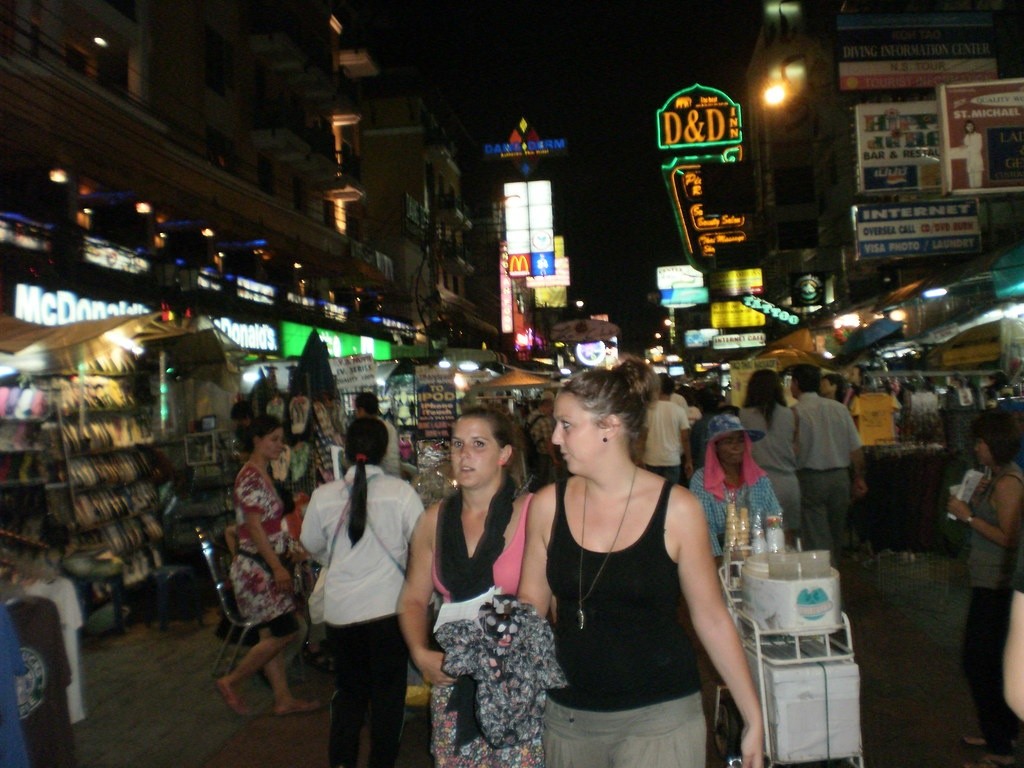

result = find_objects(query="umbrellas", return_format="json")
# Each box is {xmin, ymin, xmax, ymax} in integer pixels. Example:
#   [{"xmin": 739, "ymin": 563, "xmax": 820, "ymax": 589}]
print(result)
[
  {"xmin": 470, "ymin": 371, "xmax": 553, "ymax": 388},
  {"xmin": 839, "ymin": 318, "xmax": 904, "ymax": 354}
]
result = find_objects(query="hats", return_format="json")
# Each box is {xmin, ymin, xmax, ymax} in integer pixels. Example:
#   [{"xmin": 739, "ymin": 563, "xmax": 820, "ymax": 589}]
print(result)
[{"xmin": 706, "ymin": 415, "xmax": 765, "ymax": 443}]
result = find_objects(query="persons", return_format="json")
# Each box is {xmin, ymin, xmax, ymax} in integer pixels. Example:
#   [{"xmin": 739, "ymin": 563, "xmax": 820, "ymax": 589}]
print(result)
[
  {"xmin": 355, "ymin": 391, "xmax": 402, "ymax": 479},
  {"xmin": 215, "ymin": 413, "xmax": 325, "ymax": 714},
  {"xmin": 516, "ymin": 357, "xmax": 763, "ymax": 768},
  {"xmin": 949, "ymin": 414, "xmax": 1017, "ymax": 768},
  {"xmin": 689, "ymin": 413, "xmax": 796, "ymax": 568},
  {"xmin": 737, "ymin": 369, "xmax": 800, "ymax": 547},
  {"xmin": 790, "ymin": 364, "xmax": 868, "ymax": 570},
  {"xmin": 820, "ymin": 374, "xmax": 844, "ymax": 401},
  {"xmin": 525, "ymin": 400, "xmax": 554, "ymax": 469},
  {"xmin": 959, "ymin": 120, "xmax": 983, "ymax": 188},
  {"xmin": 396, "ymin": 403, "xmax": 556, "ymax": 768},
  {"xmin": 300, "ymin": 415, "xmax": 425, "ymax": 768},
  {"xmin": 634, "ymin": 373, "xmax": 694, "ymax": 484},
  {"xmin": 231, "ymin": 401, "xmax": 272, "ymax": 476},
  {"xmin": 384, "ymin": 400, "xmax": 417, "ymax": 426}
]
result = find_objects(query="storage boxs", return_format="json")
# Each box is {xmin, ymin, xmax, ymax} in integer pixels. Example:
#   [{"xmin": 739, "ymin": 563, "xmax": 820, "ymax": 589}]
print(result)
[{"xmin": 744, "ymin": 640, "xmax": 862, "ymax": 765}]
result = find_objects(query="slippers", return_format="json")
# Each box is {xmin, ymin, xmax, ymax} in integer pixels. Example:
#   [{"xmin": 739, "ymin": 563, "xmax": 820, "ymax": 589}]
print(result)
[
  {"xmin": 960, "ymin": 734, "xmax": 989, "ymax": 751},
  {"xmin": 963, "ymin": 751, "xmax": 1015, "ymax": 768},
  {"xmin": 274, "ymin": 698, "xmax": 322, "ymax": 715},
  {"xmin": 217, "ymin": 679, "xmax": 247, "ymax": 717}
]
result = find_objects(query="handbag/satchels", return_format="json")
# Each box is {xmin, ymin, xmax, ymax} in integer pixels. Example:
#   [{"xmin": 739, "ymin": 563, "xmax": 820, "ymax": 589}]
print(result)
[
  {"xmin": 238, "ymin": 542, "xmax": 293, "ymax": 574},
  {"xmin": 948, "ymin": 561, "xmax": 973, "ymax": 600}
]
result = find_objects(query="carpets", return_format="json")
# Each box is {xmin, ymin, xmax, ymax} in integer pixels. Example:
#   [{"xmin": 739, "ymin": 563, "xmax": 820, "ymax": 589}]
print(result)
[{"xmin": 0, "ymin": 528, "xmax": 60, "ymax": 606}]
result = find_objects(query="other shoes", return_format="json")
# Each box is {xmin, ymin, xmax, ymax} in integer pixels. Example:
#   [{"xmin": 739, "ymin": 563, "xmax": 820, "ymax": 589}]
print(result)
[
  {"xmin": 861, "ymin": 556, "xmax": 883, "ymax": 574},
  {"xmin": 898, "ymin": 553, "xmax": 918, "ymax": 568}
]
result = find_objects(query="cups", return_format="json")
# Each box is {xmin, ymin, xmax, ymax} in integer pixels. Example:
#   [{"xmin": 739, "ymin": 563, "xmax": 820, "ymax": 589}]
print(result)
[{"xmin": 745, "ymin": 549, "xmax": 832, "ymax": 581}]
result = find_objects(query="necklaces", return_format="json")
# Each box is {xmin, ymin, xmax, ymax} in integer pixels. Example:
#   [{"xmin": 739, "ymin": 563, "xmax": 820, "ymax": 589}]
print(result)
[{"xmin": 578, "ymin": 466, "xmax": 637, "ymax": 629}]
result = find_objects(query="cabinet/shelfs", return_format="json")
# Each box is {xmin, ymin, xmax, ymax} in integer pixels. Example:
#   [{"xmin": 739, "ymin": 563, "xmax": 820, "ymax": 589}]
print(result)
[{"xmin": 0, "ymin": 368, "xmax": 165, "ymax": 615}]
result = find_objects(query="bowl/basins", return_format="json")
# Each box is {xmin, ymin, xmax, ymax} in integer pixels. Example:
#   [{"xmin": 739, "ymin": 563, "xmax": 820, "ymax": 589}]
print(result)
[{"xmin": 743, "ymin": 568, "xmax": 841, "ymax": 635}]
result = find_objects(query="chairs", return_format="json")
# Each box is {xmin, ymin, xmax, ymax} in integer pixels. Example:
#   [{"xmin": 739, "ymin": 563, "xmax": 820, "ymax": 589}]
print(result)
[{"xmin": 36, "ymin": 512, "xmax": 305, "ymax": 678}]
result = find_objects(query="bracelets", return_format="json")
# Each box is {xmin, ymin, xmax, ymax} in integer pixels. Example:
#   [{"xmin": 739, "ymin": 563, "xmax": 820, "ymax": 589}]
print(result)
[{"xmin": 964, "ymin": 514, "xmax": 976, "ymax": 528}]
